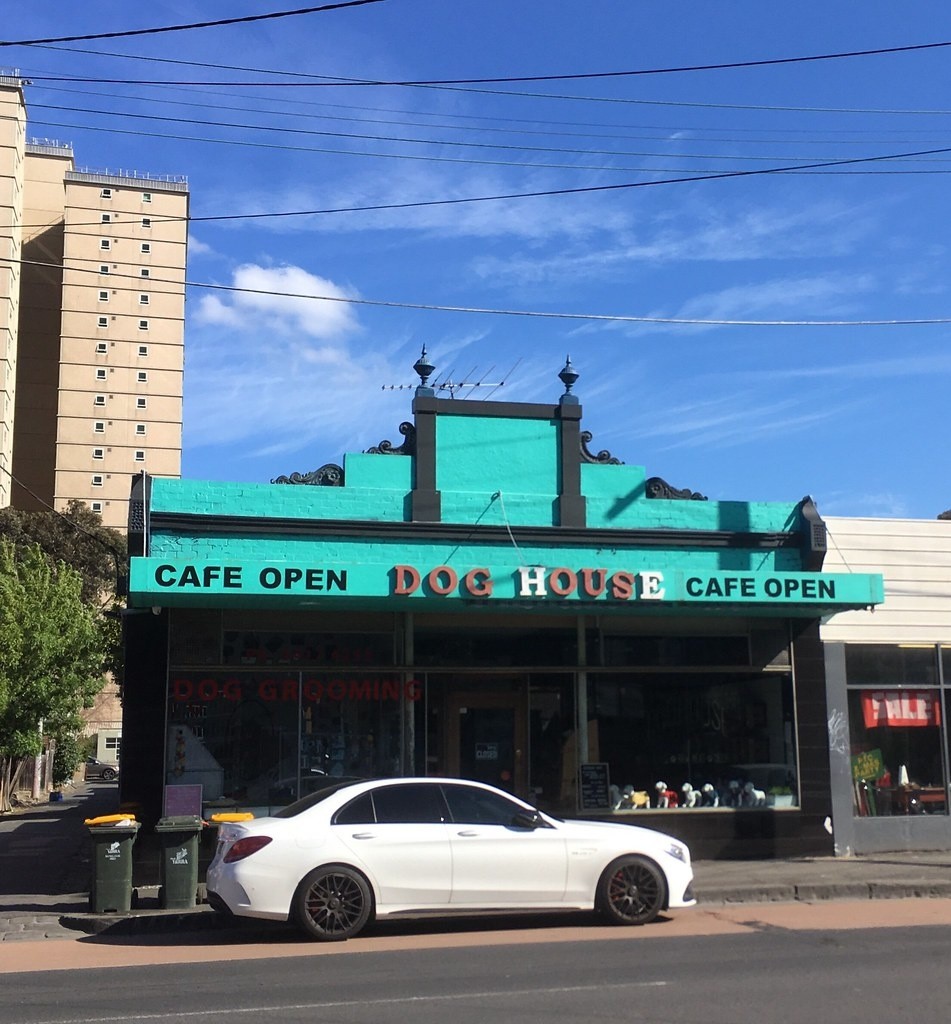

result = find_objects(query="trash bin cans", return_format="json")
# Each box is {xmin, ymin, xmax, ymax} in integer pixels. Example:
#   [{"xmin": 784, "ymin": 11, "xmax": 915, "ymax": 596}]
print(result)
[
  {"xmin": 154, "ymin": 813, "xmax": 204, "ymax": 910},
  {"xmin": 209, "ymin": 814, "xmax": 253, "ymax": 857},
  {"xmin": 83, "ymin": 815, "xmax": 141, "ymax": 915}
]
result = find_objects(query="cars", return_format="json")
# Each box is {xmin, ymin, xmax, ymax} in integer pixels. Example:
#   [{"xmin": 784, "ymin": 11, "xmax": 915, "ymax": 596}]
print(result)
[
  {"xmin": 85, "ymin": 756, "xmax": 119, "ymax": 781},
  {"xmin": 206, "ymin": 778, "xmax": 698, "ymax": 941}
]
